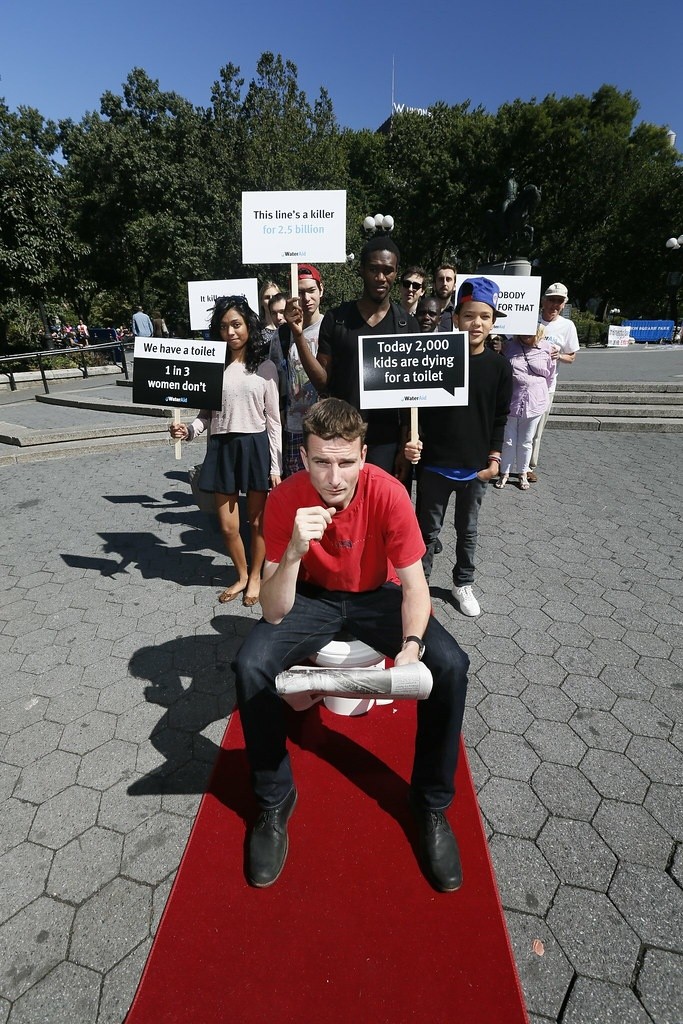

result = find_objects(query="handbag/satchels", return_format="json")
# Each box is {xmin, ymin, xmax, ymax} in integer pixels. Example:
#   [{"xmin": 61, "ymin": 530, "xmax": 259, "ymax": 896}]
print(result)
[{"xmin": 162, "ymin": 319, "xmax": 169, "ymax": 338}]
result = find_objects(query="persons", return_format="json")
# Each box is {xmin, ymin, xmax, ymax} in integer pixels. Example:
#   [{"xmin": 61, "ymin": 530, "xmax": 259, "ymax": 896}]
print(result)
[
  {"xmin": 404, "ymin": 277, "xmax": 514, "ymax": 617},
  {"xmin": 399, "ymin": 263, "xmax": 581, "ymax": 490},
  {"xmin": 283, "ymin": 236, "xmax": 404, "ymax": 495},
  {"xmin": 167, "ymin": 296, "xmax": 282, "ymax": 606},
  {"xmin": 150, "ymin": 311, "xmax": 164, "ymax": 339},
  {"xmin": 258, "ymin": 280, "xmax": 291, "ymax": 362},
  {"xmin": 234, "ymin": 397, "xmax": 470, "ymax": 892},
  {"xmin": 268, "ymin": 263, "xmax": 325, "ymax": 483},
  {"xmin": 132, "ymin": 305, "xmax": 153, "ymax": 337},
  {"xmin": 49, "ymin": 319, "xmax": 127, "ymax": 354}
]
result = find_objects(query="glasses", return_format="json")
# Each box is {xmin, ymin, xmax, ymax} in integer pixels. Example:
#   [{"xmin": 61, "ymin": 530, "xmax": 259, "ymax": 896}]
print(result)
[{"xmin": 403, "ymin": 280, "xmax": 423, "ymax": 290}]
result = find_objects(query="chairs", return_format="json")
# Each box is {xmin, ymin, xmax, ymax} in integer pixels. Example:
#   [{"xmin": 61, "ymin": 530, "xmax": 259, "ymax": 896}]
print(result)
[{"xmin": 31, "ymin": 325, "xmax": 137, "ymax": 354}]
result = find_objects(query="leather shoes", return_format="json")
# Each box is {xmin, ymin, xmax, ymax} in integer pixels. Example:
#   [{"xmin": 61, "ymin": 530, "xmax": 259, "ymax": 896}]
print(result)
[
  {"xmin": 245, "ymin": 786, "xmax": 298, "ymax": 888},
  {"xmin": 410, "ymin": 791, "xmax": 463, "ymax": 892}
]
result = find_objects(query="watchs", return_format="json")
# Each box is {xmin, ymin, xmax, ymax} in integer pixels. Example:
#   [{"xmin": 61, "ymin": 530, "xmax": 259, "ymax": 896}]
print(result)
[{"xmin": 400, "ymin": 635, "xmax": 426, "ymax": 661}]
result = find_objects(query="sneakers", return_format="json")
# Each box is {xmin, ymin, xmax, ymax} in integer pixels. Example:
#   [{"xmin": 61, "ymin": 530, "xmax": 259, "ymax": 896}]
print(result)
[{"xmin": 452, "ymin": 583, "xmax": 480, "ymax": 616}]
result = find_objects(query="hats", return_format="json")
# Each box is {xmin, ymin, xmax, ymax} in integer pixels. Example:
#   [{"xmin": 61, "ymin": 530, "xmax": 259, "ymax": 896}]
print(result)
[
  {"xmin": 134, "ymin": 306, "xmax": 144, "ymax": 312},
  {"xmin": 545, "ymin": 282, "xmax": 568, "ymax": 298},
  {"xmin": 458, "ymin": 277, "xmax": 508, "ymax": 318},
  {"xmin": 288, "ymin": 264, "xmax": 321, "ymax": 283}
]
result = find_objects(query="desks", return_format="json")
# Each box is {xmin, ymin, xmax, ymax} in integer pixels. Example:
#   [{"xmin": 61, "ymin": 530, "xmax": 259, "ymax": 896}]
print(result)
[
  {"xmin": 38, "ymin": 332, "xmax": 51, "ymax": 344},
  {"xmin": 55, "ymin": 335, "xmax": 73, "ymax": 348},
  {"xmin": 120, "ymin": 331, "xmax": 133, "ymax": 341},
  {"xmin": 624, "ymin": 319, "xmax": 675, "ymax": 343}
]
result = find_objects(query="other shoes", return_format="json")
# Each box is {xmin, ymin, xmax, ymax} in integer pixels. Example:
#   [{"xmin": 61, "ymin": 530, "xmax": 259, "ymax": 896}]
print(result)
[
  {"xmin": 527, "ymin": 472, "xmax": 537, "ymax": 481},
  {"xmin": 495, "ymin": 473, "xmax": 510, "ymax": 489},
  {"xmin": 519, "ymin": 476, "xmax": 530, "ymax": 490},
  {"xmin": 434, "ymin": 537, "xmax": 442, "ymax": 553}
]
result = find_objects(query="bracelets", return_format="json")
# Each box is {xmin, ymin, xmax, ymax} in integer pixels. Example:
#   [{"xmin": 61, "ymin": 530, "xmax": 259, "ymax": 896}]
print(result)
[
  {"xmin": 556, "ymin": 353, "xmax": 561, "ymax": 360},
  {"xmin": 488, "ymin": 454, "xmax": 501, "ymax": 464}
]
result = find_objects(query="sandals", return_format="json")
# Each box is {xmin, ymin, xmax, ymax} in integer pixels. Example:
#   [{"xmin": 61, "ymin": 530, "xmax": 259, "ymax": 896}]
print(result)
[
  {"xmin": 219, "ymin": 588, "xmax": 242, "ymax": 603},
  {"xmin": 243, "ymin": 592, "xmax": 259, "ymax": 607}
]
